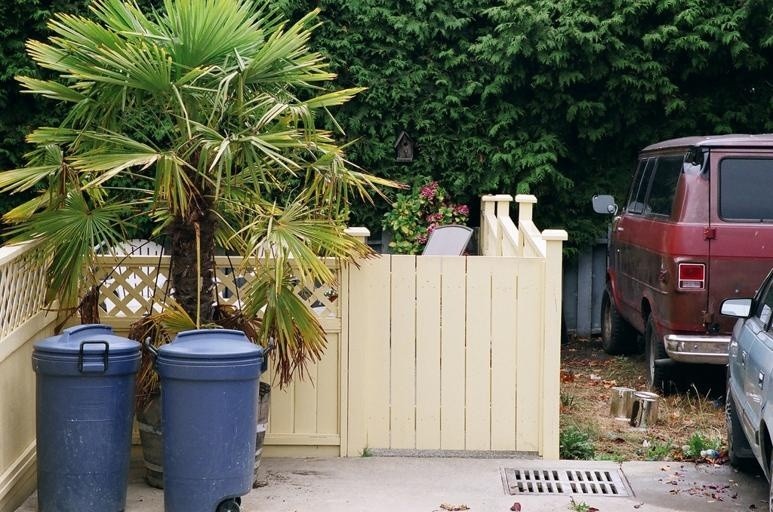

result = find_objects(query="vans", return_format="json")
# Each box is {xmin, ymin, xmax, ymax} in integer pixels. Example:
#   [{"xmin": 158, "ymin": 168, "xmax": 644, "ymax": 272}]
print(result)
[{"xmin": 590, "ymin": 131, "xmax": 771, "ymax": 388}]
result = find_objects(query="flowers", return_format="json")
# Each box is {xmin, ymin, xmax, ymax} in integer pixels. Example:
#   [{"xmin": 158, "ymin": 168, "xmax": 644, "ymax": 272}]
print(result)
[{"xmin": 381, "ymin": 175, "xmax": 470, "ymax": 257}]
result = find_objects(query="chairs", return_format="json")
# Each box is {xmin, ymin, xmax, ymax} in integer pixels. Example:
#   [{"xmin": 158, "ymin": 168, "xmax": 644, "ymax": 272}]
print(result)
[
  {"xmin": 218, "ymin": 231, "xmax": 311, "ymax": 320},
  {"xmin": 422, "ymin": 226, "xmax": 473, "ymax": 257},
  {"xmin": 99, "ymin": 239, "xmax": 174, "ymax": 317}
]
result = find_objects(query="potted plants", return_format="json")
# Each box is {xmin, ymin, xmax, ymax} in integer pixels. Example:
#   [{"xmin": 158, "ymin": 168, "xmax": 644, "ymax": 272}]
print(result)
[{"xmin": 0, "ymin": 0, "xmax": 411, "ymax": 492}]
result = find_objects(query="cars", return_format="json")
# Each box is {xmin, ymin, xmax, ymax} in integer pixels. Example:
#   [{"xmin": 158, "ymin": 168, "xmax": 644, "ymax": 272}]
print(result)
[{"xmin": 716, "ymin": 264, "xmax": 771, "ymax": 505}]
